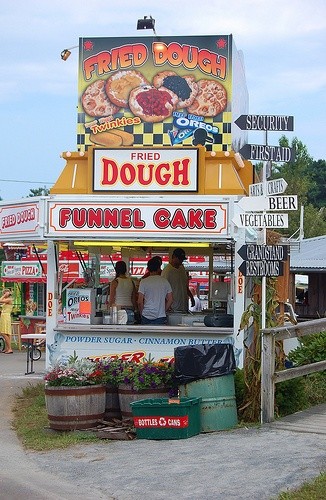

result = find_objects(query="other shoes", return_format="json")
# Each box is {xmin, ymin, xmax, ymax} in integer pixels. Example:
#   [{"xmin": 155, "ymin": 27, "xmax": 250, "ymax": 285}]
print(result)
[
  {"xmin": 2, "ymin": 350, "xmax": 7, "ymax": 353},
  {"xmin": 5, "ymin": 351, "xmax": 13, "ymax": 354}
]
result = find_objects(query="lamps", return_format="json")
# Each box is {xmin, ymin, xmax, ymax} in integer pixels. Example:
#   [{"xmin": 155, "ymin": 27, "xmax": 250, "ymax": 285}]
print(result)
[
  {"xmin": 61, "ymin": 46, "xmax": 78, "ymax": 60},
  {"xmin": 235, "ymin": 153, "xmax": 247, "ymax": 169},
  {"xmin": 137, "ymin": 15, "xmax": 156, "ymax": 35}
]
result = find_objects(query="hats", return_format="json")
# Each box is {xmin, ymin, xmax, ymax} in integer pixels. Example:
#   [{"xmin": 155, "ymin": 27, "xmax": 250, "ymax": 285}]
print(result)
[{"xmin": 173, "ymin": 248, "xmax": 188, "ymax": 261}]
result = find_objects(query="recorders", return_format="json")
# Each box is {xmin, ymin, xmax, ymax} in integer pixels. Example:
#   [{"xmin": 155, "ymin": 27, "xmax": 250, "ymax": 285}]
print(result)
[{"xmin": 204, "ymin": 308, "xmax": 234, "ymax": 328}]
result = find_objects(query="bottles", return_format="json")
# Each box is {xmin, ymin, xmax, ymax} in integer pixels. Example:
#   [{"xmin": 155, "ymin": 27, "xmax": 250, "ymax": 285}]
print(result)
[{"xmin": 97, "ymin": 294, "xmax": 109, "ymax": 311}]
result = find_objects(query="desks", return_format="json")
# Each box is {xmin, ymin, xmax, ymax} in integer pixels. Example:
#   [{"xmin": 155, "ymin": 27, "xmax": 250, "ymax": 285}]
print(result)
[{"xmin": 20, "ymin": 334, "xmax": 45, "ymax": 375}]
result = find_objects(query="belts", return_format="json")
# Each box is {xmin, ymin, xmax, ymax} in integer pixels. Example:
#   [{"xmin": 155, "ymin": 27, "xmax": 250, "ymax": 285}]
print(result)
[{"xmin": 120, "ymin": 307, "xmax": 133, "ymax": 311}]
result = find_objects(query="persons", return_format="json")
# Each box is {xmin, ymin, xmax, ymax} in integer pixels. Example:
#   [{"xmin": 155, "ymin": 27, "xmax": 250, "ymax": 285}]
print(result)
[
  {"xmin": 161, "ymin": 248, "xmax": 195, "ymax": 313},
  {"xmin": 137, "ymin": 259, "xmax": 174, "ymax": 325},
  {"xmin": 0, "ymin": 288, "xmax": 14, "ymax": 354},
  {"xmin": 110, "ymin": 261, "xmax": 136, "ymax": 325},
  {"xmin": 188, "ymin": 287, "xmax": 202, "ymax": 312},
  {"xmin": 141, "ymin": 256, "xmax": 162, "ymax": 279}
]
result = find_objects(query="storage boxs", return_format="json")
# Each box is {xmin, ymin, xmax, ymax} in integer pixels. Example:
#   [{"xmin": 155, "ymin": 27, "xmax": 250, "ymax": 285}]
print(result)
[{"xmin": 130, "ymin": 397, "xmax": 202, "ymax": 440}]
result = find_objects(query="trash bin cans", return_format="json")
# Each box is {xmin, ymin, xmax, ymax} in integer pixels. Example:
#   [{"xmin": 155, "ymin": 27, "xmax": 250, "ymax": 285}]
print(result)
[{"xmin": 174, "ymin": 344, "xmax": 238, "ymax": 433}]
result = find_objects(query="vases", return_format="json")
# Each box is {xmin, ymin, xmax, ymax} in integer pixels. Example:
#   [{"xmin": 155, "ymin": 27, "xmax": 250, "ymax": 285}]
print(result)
[
  {"xmin": 44, "ymin": 385, "xmax": 106, "ymax": 430},
  {"xmin": 118, "ymin": 382, "xmax": 172, "ymax": 426},
  {"xmin": 103, "ymin": 383, "xmax": 120, "ymax": 418}
]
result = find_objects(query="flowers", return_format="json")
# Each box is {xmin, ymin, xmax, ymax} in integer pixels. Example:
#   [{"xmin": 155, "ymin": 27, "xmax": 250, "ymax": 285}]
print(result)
[{"xmin": 44, "ymin": 354, "xmax": 179, "ymax": 398}]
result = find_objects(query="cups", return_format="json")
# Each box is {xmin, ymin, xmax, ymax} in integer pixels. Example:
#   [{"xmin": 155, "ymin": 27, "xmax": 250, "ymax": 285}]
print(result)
[{"xmin": 103, "ymin": 307, "xmax": 127, "ymax": 325}]
